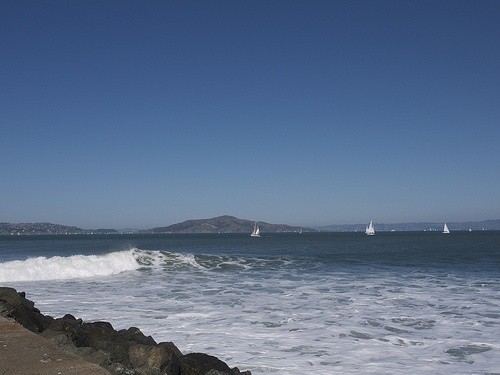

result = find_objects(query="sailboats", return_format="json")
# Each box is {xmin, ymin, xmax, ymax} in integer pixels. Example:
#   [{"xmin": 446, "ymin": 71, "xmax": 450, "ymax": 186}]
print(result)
[
  {"xmin": 250, "ymin": 221, "xmax": 261, "ymax": 238},
  {"xmin": 441, "ymin": 223, "xmax": 451, "ymax": 234},
  {"xmin": 365, "ymin": 218, "xmax": 375, "ymax": 236}
]
